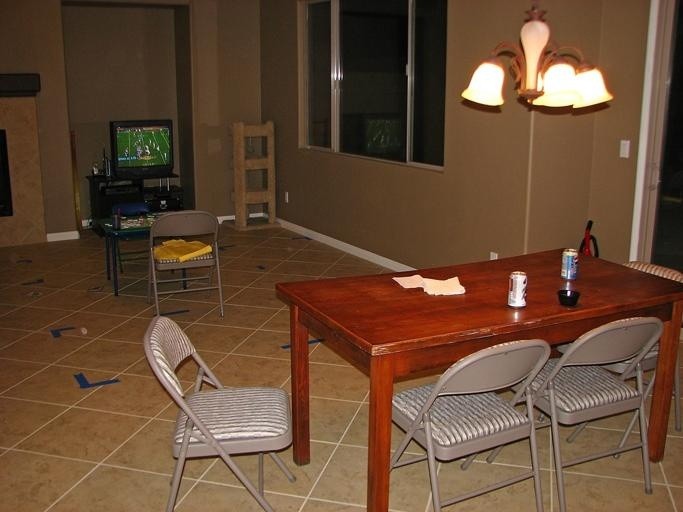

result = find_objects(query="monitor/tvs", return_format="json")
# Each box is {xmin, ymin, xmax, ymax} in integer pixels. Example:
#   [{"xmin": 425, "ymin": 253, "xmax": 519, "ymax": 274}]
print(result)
[
  {"xmin": 358, "ymin": 114, "xmax": 407, "ymax": 159},
  {"xmin": 109, "ymin": 118, "xmax": 174, "ymax": 179}
]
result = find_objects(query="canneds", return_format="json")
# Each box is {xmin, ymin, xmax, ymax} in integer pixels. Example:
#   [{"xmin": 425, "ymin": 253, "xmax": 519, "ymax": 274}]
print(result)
[
  {"xmin": 560, "ymin": 248, "xmax": 579, "ymax": 279},
  {"xmin": 507, "ymin": 271, "xmax": 528, "ymax": 309}
]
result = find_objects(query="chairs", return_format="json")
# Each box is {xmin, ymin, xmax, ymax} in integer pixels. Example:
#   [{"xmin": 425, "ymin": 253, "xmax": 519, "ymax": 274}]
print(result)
[
  {"xmin": 140, "ymin": 315, "xmax": 297, "ymax": 511},
  {"xmin": 389, "ymin": 339, "xmax": 551, "ymax": 512},
  {"xmin": 111, "ymin": 202, "xmax": 152, "ymax": 273},
  {"xmin": 621, "ymin": 261, "xmax": 683, "ymax": 284},
  {"xmin": 485, "ymin": 317, "xmax": 663, "ymax": 511},
  {"xmin": 146, "ymin": 211, "xmax": 225, "ymax": 317},
  {"xmin": 551, "ymin": 262, "xmax": 683, "ymax": 459}
]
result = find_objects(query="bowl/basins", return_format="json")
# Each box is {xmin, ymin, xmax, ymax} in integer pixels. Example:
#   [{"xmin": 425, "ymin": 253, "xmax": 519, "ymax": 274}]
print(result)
[{"xmin": 557, "ymin": 289, "xmax": 580, "ymax": 307}]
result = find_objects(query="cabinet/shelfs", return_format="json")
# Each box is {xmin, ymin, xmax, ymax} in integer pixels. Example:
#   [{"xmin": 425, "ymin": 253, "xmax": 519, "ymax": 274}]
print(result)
[
  {"xmin": 229, "ymin": 119, "xmax": 280, "ymax": 231},
  {"xmin": 85, "ymin": 173, "xmax": 185, "ymax": 238}
]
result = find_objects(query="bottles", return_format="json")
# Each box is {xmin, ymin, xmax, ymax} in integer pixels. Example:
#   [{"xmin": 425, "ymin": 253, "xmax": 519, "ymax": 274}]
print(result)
[{"xmin": 92, "ymin": 163, "xmax": 98, "ymax": 175}]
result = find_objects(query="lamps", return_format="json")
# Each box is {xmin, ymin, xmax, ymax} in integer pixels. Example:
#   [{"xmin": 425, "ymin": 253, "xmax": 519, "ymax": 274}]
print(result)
[{"xmin": 459, "ymin": 1, "xmax": 614, "ymax": 256}]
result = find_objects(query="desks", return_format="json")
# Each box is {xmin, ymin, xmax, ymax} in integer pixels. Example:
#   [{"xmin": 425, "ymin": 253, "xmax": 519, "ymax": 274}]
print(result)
[
  {"xmin": 95, "ymin": 213, "xmax": 187, "ymax": 297},
  {"xmin": 274, "ymin": 247, "xmax": 683, "ymax": 511}
]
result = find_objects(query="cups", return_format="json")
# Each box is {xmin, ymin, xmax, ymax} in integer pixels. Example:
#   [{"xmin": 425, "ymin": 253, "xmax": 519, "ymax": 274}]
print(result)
[{"xmin": 111, "ymin": 214, "xmax": 120, "ymax": 231}]
